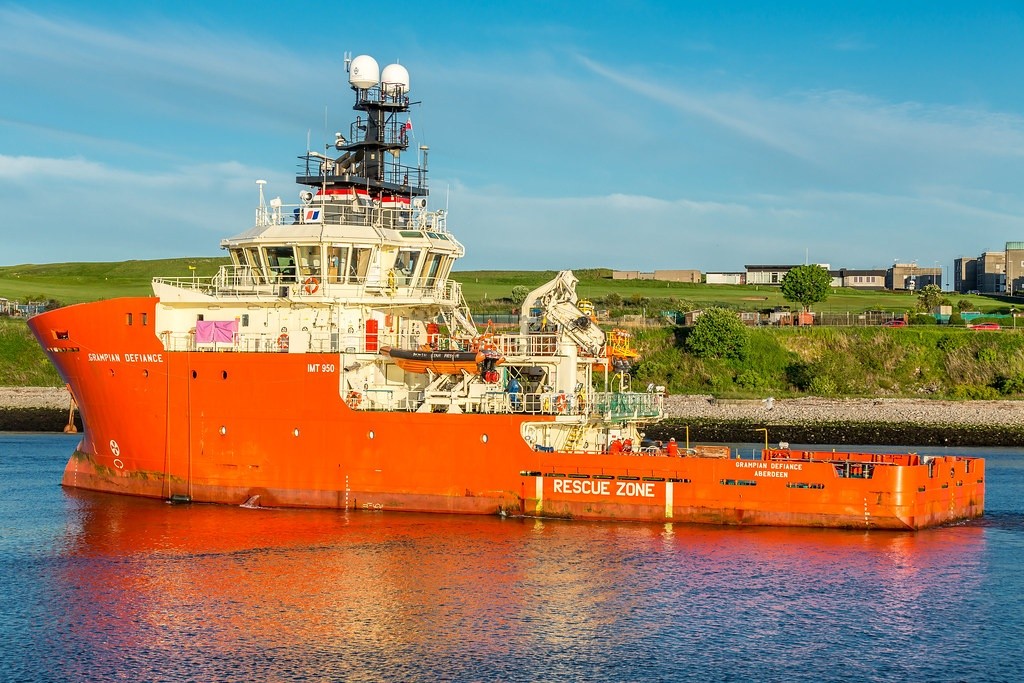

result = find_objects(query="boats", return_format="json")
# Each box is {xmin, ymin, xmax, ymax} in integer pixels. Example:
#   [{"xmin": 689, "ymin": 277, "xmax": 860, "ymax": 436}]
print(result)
[
  {"xmin": 389, "ymin": 346, "xmax": 507, "ymax": 376},
  {"xmin": 26, "ymin": 51, "xmax": 987, "ymax": 534}
]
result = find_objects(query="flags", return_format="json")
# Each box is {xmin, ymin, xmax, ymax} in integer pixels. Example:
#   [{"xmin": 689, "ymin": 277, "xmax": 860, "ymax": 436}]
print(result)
[{"xmin": 398, "ymin": 116, "xmax": 412, "ymax": 139}]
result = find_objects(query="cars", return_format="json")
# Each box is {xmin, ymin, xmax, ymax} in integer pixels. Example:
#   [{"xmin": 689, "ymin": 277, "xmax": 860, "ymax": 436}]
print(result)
[
  {"xmin": 970, "ymin": 321, "xmax": 1000, "ymax": 330},
  {"xmin": 882, "ymin": 321, "xmax": 905, "ymax": 328}
]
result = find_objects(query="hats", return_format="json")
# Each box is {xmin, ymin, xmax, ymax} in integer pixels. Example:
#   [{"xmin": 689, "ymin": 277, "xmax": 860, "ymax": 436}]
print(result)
[
  {"xmin": 669, "ymin": 438, "xmax": 676, "ymax": 442},
  {"xmin": 543, "ymin": 386, "xmax": 548, "ymax": 390},
  {"xmin": 617, "ymin": 436, "xmax": 622, "ymax": 440},
  {"xmin": 629, "ymin": 437, "xmax": 634, "ymax": 439}
]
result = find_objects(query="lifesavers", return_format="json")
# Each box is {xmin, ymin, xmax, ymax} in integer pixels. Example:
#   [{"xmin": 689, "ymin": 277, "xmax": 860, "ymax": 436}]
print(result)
[
  {"xmin": 556, "ymin": 395, "xmax": 567, "ymax": 412},
  {"xmin": 305, "ymin": 277, "xmax": 319, "ymax": 293},
  {"xmin": 277, "ymin": 333, "xmax": 289, "ymax": 349}
]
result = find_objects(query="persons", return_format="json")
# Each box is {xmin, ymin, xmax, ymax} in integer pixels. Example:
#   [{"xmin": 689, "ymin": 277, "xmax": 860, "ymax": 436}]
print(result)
[
  {"xmin": 609, "ymin": 436, "xmax": 634, "ymax": 456},
  {"xmin": 282, "ymin": 260, "xmax": 296, "ymax": 281},
  {"xmin": 427, "ymin": 319, "xmax": 438, "ymax": 351},
  {"xmin": 485, "ymin": 369, "xmax": 499, "ymax": 384},
  {"xmin": 666, "ymin": 437, "xmax": 678, "ymax": 458}
]
